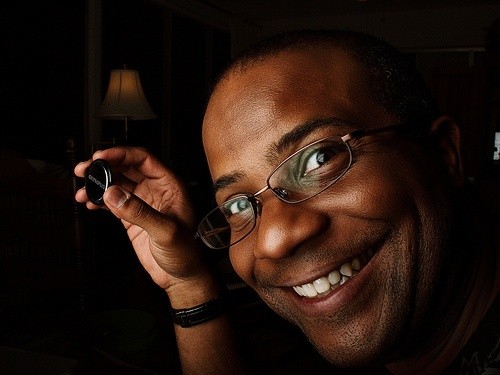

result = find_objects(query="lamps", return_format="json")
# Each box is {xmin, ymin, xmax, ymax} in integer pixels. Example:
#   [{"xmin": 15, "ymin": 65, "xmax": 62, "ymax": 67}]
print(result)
[{"xmin": 94, "ymin": 64, "xmax": 158, "ymax": 145}]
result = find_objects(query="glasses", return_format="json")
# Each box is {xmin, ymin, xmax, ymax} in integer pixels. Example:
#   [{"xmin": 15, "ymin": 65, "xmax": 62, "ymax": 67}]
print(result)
[{"xmin": 195, "ymin": 116, "xmax": 435, "ymax": 249}]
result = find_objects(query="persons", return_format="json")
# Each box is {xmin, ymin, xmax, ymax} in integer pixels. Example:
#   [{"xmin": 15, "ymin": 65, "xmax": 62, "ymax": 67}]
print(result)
[{"xmin": 74, "ymin": 28, "xmax": 500, "ymax": 375}]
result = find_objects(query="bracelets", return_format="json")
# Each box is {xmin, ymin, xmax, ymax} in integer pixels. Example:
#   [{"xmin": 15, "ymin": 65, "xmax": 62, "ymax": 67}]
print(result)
[{"xmin": 169, "ymin": 285, "xmax": 224, "ymax": 327}]
